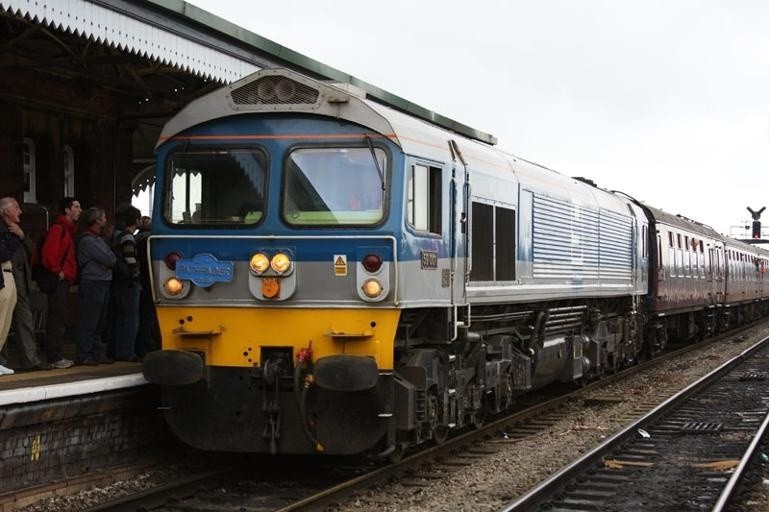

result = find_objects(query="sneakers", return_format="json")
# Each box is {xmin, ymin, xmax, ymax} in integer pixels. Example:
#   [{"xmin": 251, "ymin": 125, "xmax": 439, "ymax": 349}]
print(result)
[
  {"xmin": 0, "ymin": 364, "xmax": 15, "ymax": 376},
  {"xmin": 76, "ymin": 355, "xmax": 114, "ymax": 365},
  {"xmin": 42, "ymin": 358, "xmax": 76, "ymax": 370}
]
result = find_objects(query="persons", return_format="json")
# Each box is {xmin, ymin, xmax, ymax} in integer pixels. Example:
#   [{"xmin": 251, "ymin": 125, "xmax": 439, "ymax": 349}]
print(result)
[{"xmin": 0, "ymin": 196, "xmax": 162, "ymax": 377}]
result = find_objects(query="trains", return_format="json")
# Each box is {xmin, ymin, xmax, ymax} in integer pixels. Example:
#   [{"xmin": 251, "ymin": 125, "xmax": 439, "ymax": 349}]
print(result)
[{"xmin": 143, "ymin": 66, "xmax": 769, "ymax": 473}]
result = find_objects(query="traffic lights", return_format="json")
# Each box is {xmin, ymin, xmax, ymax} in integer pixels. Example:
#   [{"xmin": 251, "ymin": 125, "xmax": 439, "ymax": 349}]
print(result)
[{"xmin": 753, "ymin": 221, "xmax": 761, "ymax": 238}]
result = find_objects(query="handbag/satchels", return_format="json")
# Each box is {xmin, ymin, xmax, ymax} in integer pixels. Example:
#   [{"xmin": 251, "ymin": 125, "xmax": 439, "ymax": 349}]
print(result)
[{"xmin": 36, "ymin": 269, "xmax": 59, "ymax": 295}]
